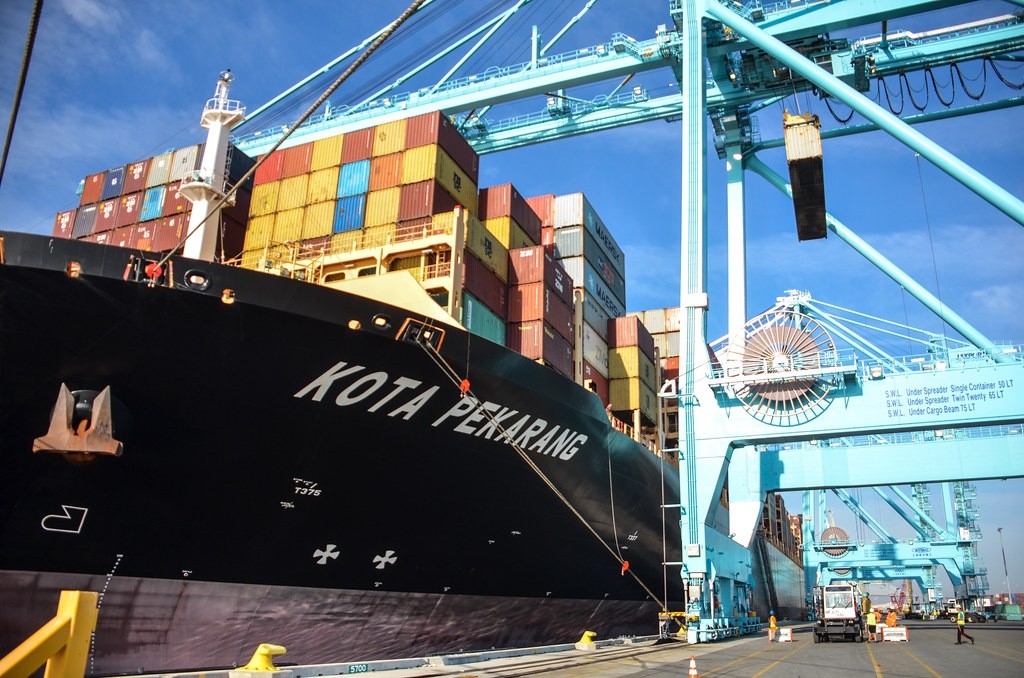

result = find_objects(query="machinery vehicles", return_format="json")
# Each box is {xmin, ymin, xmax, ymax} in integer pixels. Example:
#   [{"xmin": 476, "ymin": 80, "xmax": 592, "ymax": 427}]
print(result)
[{"xmin": 811, "ymin": 582, "xmax": 864, "ymax": 642}]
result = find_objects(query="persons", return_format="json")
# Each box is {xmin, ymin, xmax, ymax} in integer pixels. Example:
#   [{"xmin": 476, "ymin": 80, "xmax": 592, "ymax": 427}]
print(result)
[
  {"xmin": 874, "ymin": 608, "xmax": 881, "ymax": 623},
  {"xmin": 769, "ymin": 610, "xmax": 778, "ymax": 640},
  {"xmin": 865, "ymin": 609, "xmax": 878, "ymax": 642},
  {"xmin": 861, "ymin": 592, "xmax": 871, "ymax": 615},
  {"xmin": 921, "ymin": 609, "xmax": 940, "ymax": 620},
  {"xmin": 953, "ymin": 608, "xmax": 974, "ymax": 646},
  {"xmin": 886, "ymin": 609, "xmax": 897, "ymax": 627}
]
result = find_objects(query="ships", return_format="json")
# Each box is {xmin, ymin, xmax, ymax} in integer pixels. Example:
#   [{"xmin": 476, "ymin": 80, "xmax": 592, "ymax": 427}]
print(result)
[{"xmin": 0, "ymin": 68, "xmax": 807, "ymax": 678}]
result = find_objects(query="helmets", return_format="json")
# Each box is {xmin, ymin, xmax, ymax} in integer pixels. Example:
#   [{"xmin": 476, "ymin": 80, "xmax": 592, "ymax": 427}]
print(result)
[
  {"xmin": 769, "ymin": 610, "xmax": 774, "ymax": 615},
  {"xmin": 869, "ymin": 608, "xmax": 874, "ymax": 612},
  {"xmin": 955, "ymin": 604, "xmax": 961, "ymax": 607},
  {"xmin": 864, "ymin": 593, "xmax": 869, "ymax": 596},
  {"xmin": 889, "ymin": 606, "xmax": 894, "ymax": 609}
]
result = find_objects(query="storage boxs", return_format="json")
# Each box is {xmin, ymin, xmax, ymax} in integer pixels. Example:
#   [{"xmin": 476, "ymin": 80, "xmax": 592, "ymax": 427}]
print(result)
[
  {"xmin": 761, "ymin": 492, "xmax": 802, "ymax": 559},
  {"xmin": 782, "ymin": 111, "xmax": 826, "ymax": 241},
  {"xmin": 55, "ymin": 109, "xmax": 680, "ymax": 440},
  {"xmin": 984, "ymin": 593, "xmax": 1024, "ymax": 622}
]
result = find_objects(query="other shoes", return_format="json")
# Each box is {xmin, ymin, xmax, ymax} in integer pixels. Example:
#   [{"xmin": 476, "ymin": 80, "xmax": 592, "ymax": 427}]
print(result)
[
  {"xmin": 971, "ymin": 637, "xmax": 974, "ymax": 644},
  {"xmin": 954, "ymin": 642, "xmax": 962, "ymax": 645}
]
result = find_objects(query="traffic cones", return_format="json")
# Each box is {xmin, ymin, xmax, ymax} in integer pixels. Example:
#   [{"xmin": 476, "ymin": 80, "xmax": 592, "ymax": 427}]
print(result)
[{"xmin": 688, "ymin": 656, "xmax": 700, "ymax": 678}]
